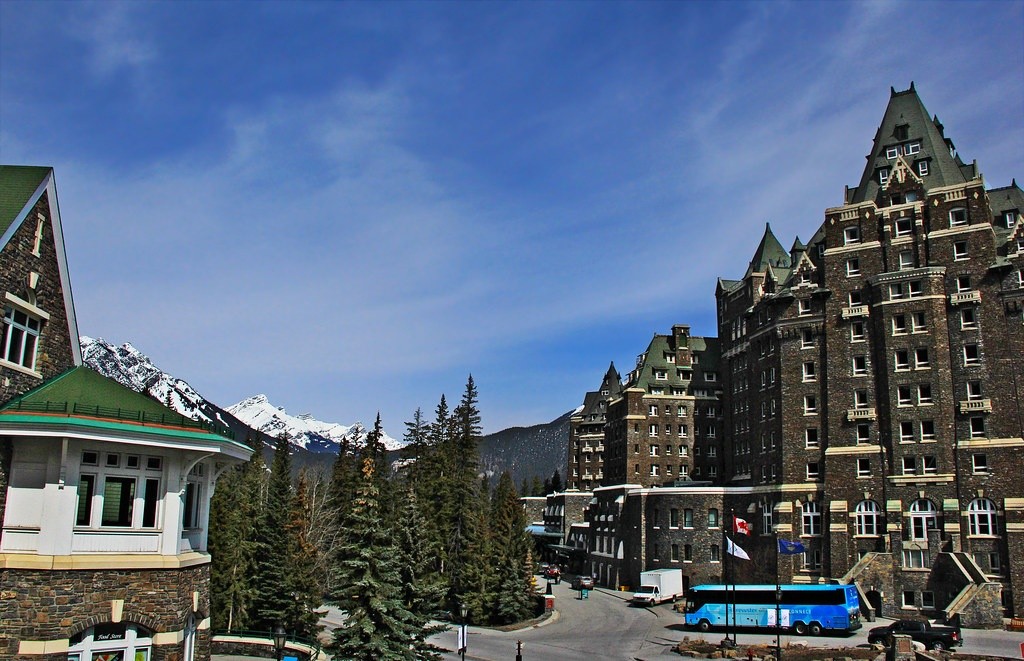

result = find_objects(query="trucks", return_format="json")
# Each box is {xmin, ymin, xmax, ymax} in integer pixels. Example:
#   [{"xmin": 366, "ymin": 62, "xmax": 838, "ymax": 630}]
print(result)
[{"xmin": 632, "ymin": 568, "xmax": 684, "ymax": 606}]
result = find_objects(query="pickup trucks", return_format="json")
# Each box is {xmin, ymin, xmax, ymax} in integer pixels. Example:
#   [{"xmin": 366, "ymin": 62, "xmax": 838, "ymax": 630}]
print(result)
[{"xmin": 867, "ymin": 618, "xmax": 963, "ymax": 650}]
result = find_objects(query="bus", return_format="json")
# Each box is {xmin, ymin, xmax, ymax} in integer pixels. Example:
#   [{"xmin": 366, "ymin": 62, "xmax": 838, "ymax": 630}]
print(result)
[{"xmin": 684, "ymin": 584, "xmax": 863, "ymax": 636}]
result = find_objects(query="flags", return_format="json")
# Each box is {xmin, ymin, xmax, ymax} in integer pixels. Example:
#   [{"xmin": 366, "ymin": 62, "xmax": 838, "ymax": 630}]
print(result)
[
  {"xmin": 778, "ymin": 539, "xmax": 806, "ymax": 554},
  {"xmin": 725, "ymin": 536, "xmax": 751, "ymax": 560},
  {"xmin": 733, "ymin": 517, "xmax": 750, "ymax": 536}
]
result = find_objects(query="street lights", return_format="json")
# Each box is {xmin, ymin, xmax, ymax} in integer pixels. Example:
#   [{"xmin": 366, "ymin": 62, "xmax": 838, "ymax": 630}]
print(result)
[
  {"xmin": 775, "ymin": 587, "xmax": 782, "ymax": 661},
  {"xmin": 273, "ymin": 624, "xmax": 286, "ymax": 661},
  {"xmin": 459, "ymin": 603, "xmax": 468, "ymax": 661}
]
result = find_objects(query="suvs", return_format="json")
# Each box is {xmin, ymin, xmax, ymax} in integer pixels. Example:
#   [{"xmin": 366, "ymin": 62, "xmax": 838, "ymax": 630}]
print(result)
[
  {"xmin": 544, "ymin": 565, "xmax": 561, "ymax": 578},
  {"xmin": 538, "ymin": 563, "xmax": 550, "ymax": 574},
  {"xmin": 572, "ymin": 575, "xmax": 594, "ymax": 591}
]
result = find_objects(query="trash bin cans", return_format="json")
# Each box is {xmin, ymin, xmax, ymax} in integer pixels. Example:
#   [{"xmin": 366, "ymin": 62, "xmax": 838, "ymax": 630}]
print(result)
[{"xmin": 279, "ymin": 650, "xmax": 299, "ymax": 661}]
